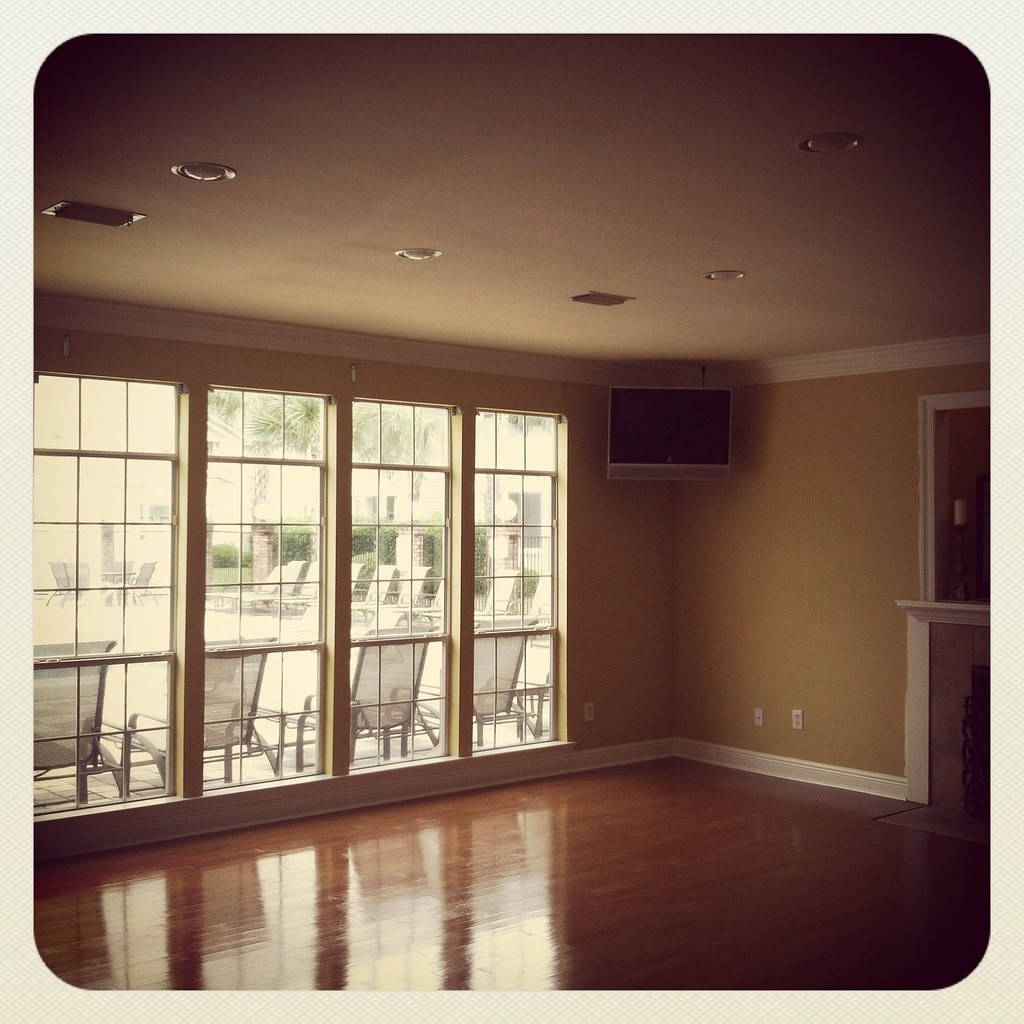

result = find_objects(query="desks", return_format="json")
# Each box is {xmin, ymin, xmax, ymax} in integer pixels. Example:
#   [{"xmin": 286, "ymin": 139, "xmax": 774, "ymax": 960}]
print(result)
[{"xmin": 99, "ymin": 571, "xmax": 136, "ymax": 607}]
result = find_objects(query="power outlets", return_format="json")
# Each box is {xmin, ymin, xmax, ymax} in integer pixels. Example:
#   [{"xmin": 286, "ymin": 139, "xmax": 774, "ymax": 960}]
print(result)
[
  {"xmin": 584, "ymin": 702, "xmax": 593, "ymax": 721},
  {"xmin": 754, "ymin": 708, "xmax": 763, "ymax": 726},
  {"xmin": 792, "ymin": 709, "xmax": 802, "ymax": 729}
]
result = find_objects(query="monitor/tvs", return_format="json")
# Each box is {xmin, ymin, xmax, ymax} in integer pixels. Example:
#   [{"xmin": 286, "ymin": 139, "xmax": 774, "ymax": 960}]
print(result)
[{"xmin": 607, "ymin": 386, "xmax": 732, "ymax": 480}]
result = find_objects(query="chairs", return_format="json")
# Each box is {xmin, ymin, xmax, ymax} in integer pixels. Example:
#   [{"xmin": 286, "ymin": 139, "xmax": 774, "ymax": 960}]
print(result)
[
  {"xmin": 273, "ymin": 563, "xmax": 365, "ymax": 618},
  {"xmin": 33, "ymin": 641, "xmax": 132, "ymax": 806},
  {"xmin": 43, "ymin": 559, "xmax": 160, "ymax": 609},
  {"xmin": 351, "ymin": 566, "xmax": 431, "ymax": 627},
  {"xmin": 82, "ymin": 637, "xmax": 285, "ymax": 785},
  {"xmin": 418, "ymin": 568, "xmax": 521, "ymax": 629},
  {"xmin": 242, "ymin": 562, "xmax": 319, "ymax": 616},
  {"xmin": 375, "ymin": 579, "xmax": 444, "ymax": 626},
  {"xmin": 204, "ymin": 565, "xmax": 287, "ymax": 611},
  {"xmin": 306, "ymin": 564, "xmax": 397, "ymax": 624},
  {"xmin": 295, "ymin": 635, "xmax": 429, "ymax": 773},
  {"xmin": 382, "ymin": 625, "xmax": 545, "ymax": 760},
  {"xmin": 474, "ymin": 576, "xmax": 551, "ymax": 649},
  {"xmin": 215, "ymin": 560, "xmax": 307, "ymax": 615}
]
